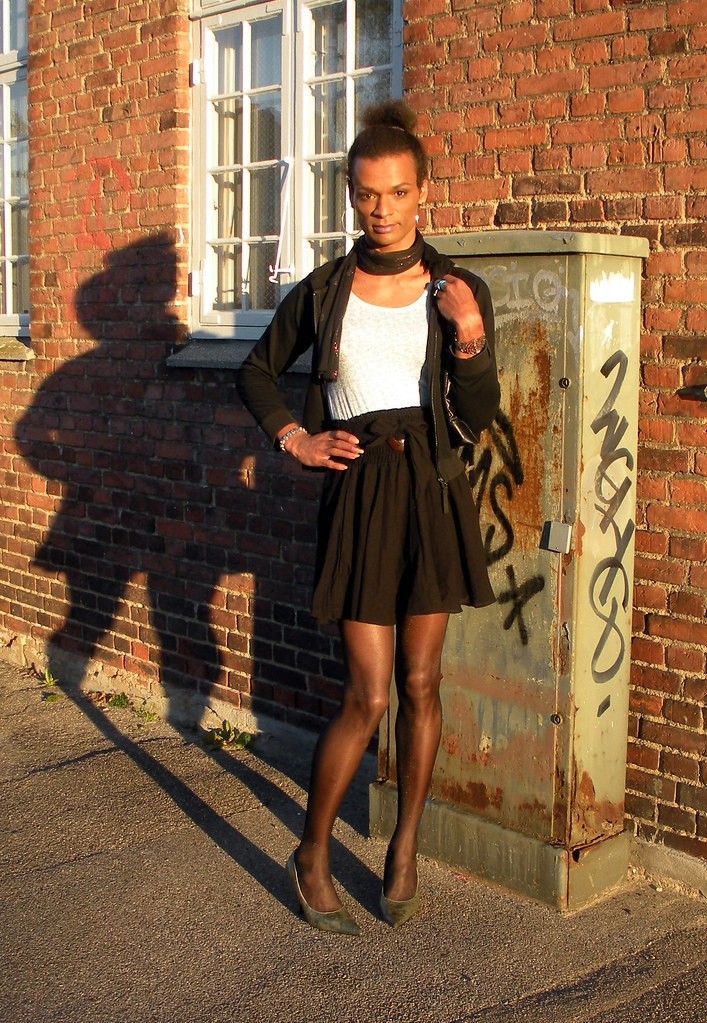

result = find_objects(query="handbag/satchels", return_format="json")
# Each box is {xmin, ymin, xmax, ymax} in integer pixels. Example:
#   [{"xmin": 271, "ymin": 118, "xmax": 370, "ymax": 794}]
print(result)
[{"xmin": 441, "ymin": 371, "xmax": 479, "ymax": 441}]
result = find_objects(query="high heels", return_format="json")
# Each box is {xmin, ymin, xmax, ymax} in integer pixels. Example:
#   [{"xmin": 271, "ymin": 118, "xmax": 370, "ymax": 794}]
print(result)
[
  {"xmin": 285, "ymin": 847, "xmax": 363, "ymax": 935},
  {"xmin": 380, "ymin": 867, "xmax": 419, "ymax": 928}
]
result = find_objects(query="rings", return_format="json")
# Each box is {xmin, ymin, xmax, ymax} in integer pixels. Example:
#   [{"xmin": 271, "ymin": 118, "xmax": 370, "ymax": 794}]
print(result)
[
  {"xmin": 433, "ymin": 289, "xmax": 439, "ymax": 296},
  {"xmin": 437, "ymin": 279, "xmax": 446, "ymax": 290}
]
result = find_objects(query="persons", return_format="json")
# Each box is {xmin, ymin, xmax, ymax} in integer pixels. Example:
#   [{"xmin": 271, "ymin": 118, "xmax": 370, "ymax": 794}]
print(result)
[{"xmin": 236, "ymin": 101, "xmax": 501, "ymax": 937}]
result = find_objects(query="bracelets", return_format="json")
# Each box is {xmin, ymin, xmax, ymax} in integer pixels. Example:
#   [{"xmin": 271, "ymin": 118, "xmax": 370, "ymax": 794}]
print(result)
[
  {"xmin": 454, "ymin": 334, "xmax": 486, "ymax": 354},
  {"xmin": 280, "ymin": 427, "xmax": 308, "ymax": 453}
]
